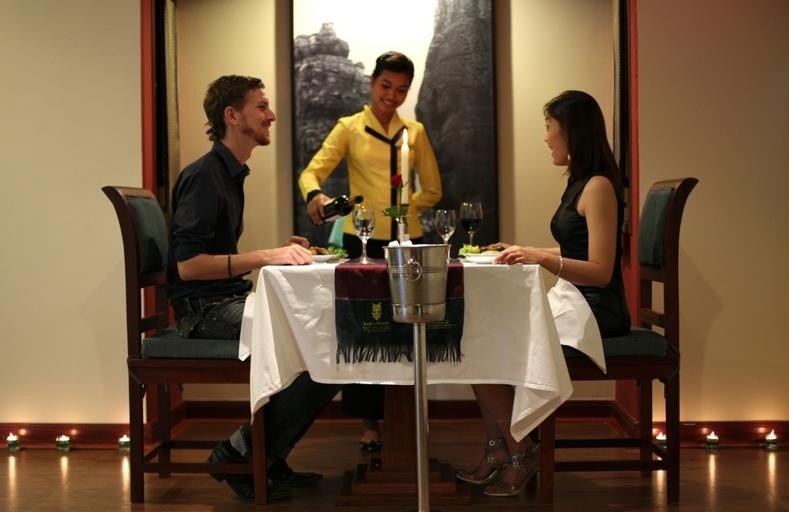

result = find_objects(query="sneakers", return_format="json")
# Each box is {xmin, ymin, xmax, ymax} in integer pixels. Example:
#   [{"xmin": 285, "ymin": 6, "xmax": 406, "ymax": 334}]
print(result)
[{"xmin": 359, "ymin": 432, "xmax": 383, "ymax": 455}]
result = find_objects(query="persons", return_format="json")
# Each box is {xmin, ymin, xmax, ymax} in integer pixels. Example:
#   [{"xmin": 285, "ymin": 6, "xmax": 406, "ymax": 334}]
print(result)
[
  {"xmin": 454, "ymin": 90, "xmax": 631, "ymax": 498},
  {"xmin": 297, "ymin": 51, "xmax": 443, "ymax": 461},
  {"xmin": 166, "ymin": 76, "xmax": 342, "ymax": 500}
]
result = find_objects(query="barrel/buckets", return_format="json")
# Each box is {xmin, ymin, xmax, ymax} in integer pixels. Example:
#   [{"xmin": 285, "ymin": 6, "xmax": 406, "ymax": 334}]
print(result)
[{"xmin": 382, "ymin": 244, "xmax": 449, "ymax": 324}]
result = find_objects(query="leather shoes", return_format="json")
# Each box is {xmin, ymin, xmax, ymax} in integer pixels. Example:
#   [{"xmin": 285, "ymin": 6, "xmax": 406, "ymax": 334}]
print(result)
[
  {"xmin": 262, "ymin": 460, "xmax": 322, "ymax": 488},
  {"xmin": 205, "ymin": 439, "xmax": 291, "ymax": 503}
]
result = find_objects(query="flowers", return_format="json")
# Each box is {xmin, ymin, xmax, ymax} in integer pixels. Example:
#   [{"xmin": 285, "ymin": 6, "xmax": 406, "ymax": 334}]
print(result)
[{"xmin": 385, "ymin": 173, "xmax": 414, "ymax": 221}]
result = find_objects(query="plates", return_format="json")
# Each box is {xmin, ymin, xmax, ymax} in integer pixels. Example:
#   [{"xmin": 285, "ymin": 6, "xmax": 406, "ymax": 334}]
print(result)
[
  {"xmin": 465, "ymin": 253, "xmax": 503, "ymax": 265},
  {"xmin": 308, "ymin": 252, "xmax": 339, "ymax": 263}
]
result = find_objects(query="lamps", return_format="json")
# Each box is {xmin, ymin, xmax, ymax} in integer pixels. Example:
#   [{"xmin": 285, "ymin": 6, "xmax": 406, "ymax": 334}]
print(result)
[
  {"xmin": 654, "ymin": 427, "xmax": 781, "ymax": 452},
  {"xmin": 4, "ymin": 429, "xmax": 132, "ymax": 457}
]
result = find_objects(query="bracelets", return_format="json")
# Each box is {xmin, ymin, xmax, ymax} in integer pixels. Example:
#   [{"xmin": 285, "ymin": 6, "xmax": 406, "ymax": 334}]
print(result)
[
  {"xmin": 555, "ymin": 256, "xmax": 564, "ymax": 279},
  {"xmin": 226, "ymin": 252, "xmax": 234, "ymax": 279}
]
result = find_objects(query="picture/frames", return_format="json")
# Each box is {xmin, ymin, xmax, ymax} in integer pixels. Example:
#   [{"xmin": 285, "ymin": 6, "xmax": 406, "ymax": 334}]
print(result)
[{"xmin": 286, "ymin": 0, "xmax": 503, "ymax": 250}]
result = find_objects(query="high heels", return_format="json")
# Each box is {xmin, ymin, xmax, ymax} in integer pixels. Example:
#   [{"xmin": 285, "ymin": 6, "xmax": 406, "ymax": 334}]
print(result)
[
  {"xmin": 483, "ymin": 443, "xmax": 541, "ymax": 498},
  {"xmin": 456, "ymin": 439, "xmax": 510, "ymax": 484}
]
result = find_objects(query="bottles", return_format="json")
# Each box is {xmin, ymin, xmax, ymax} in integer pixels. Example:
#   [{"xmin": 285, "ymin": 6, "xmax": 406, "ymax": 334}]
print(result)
[{"xmin": 312, "ymin": 193, "xmax": 363, "ymax": 226}]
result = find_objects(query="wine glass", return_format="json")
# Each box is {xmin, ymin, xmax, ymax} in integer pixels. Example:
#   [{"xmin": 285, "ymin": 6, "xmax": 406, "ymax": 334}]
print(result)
[
  {"xmin": 433, "ymin": 206, "xmax": 458, "ymax": 258},
  {"xmin": 349, "ymin": 203, "xmax": 378, "ymax": 266},
  {"xmin": 460, "ymin": 199, "xmax": 484, "ymax": 253}
]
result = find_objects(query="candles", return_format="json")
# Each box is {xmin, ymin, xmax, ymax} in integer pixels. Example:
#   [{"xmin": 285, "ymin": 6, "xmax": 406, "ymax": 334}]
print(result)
[{"xmin": 397, "ymin": 127, "xmax": 413, "ymax": 208}]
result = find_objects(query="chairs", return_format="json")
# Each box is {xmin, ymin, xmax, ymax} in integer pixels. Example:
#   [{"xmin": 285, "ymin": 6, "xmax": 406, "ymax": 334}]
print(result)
[
  {"xmin": 97, "ymin": 184, "xmax": 281, "ymax": 506},
  {"xmin": 537, "ymin": 172, "xmax": 705, "ymax": 509}
]
